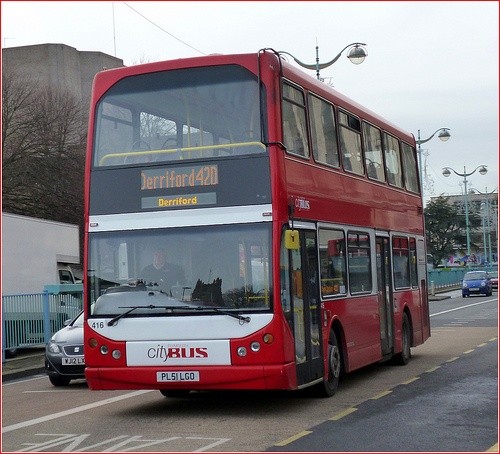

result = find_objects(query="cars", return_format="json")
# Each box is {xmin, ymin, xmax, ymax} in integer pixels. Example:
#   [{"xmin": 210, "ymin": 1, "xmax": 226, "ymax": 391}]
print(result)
[
  {"xmin": 460, "ymin": 270, "xmax": 492, "ymax": 298},
  {"xmin": 45, "ymin": 309, "xmax": 85, "ymax": 387},
  {"xmin": 489, "ymin": 271, "xmax": 498, "ymax": 289}
]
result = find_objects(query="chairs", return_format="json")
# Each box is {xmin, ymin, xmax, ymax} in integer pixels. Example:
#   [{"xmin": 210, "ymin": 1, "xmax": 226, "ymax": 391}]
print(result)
[
  {"xmin": 349, "ymin": 154, "xmax": 390, "ymax": 184},
  {"xmin": 332, "ymin": 256, "xmax": 408, "ymax": 293},
  {"xmin": 124, "ymin": 138, "xmax": 178, "ymax": 163}
]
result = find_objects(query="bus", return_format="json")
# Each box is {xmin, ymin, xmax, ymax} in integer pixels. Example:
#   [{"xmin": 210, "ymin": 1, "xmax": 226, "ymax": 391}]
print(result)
[{"xmin": 84, "ymin": 48, "xmax": 431, "ymax": 398}]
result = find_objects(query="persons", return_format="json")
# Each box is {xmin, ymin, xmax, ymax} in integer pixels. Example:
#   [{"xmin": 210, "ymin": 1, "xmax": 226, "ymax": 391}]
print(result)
[{"xmin": 140, "ymin": 248, "xmax": 186, "ymax": 293}]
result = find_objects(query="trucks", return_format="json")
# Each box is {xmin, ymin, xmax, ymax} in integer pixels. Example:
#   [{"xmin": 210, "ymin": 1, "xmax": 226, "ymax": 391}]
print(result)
[{"xmin": 1, "ymin": 212, "xmax": 83, "ymax": 357}]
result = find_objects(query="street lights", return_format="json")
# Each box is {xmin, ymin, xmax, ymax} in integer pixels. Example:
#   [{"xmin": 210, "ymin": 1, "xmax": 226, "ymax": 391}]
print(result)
[
  {"xmin": 272, "ymin": 42, "xmax": 369, "ymax": 82},
  {"xmin": 441, "ymin": 164, "xmax": 489, "ymax": 268},
  {"xmin": 468, "ymin": 185, "xmax": 498, "ymax": 267},
  {"xmin": 415, "ymin": 127, "xmax": 451, "ymax": 189}
]
